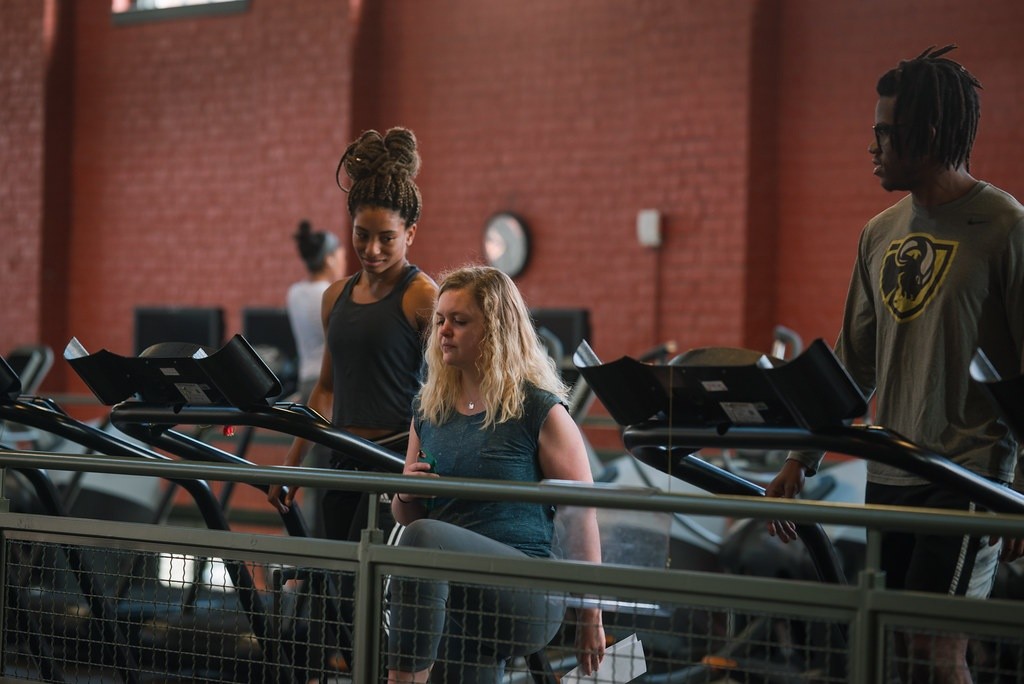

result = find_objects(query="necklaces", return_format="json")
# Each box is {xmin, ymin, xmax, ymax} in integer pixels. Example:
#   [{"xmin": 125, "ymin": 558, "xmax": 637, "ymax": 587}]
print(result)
[{"xmin": 464, "ymin": 397, "xmax": 481, "ymax": 412}]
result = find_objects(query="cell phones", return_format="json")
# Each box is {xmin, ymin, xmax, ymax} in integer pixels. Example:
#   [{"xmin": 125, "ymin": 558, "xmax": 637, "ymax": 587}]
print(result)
[{"xmin": 416, "ymin": 446, "xmax": 436, "ymax": 496}]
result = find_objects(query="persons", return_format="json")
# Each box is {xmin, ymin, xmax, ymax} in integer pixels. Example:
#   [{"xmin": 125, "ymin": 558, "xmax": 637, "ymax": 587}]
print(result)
[
  {"xmin": 285, "ymin": 218, "xmax": 348, "ymax": 409},
  {"xmin": 764, "ymin": 43, "xmax": 1024, "ymax": 684},
  {"xmin": 382, "ymin": 264, "xmax": 607, "ymax": 684},
  {"xmin": 267, "ymin": 127, "xmax": 441, "ymax": 682}
]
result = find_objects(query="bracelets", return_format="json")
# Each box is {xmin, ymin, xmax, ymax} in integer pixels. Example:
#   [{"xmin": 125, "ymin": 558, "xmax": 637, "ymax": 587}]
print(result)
[{"xmin": 397, "ymin": 493, "xmax": 413, "ymax": 504}]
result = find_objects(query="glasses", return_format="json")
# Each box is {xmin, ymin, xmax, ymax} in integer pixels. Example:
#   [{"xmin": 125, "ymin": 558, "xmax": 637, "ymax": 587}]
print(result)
[{"xmin": 873, "ymin": 123, "xmax": 934, "ymax": 151}]
